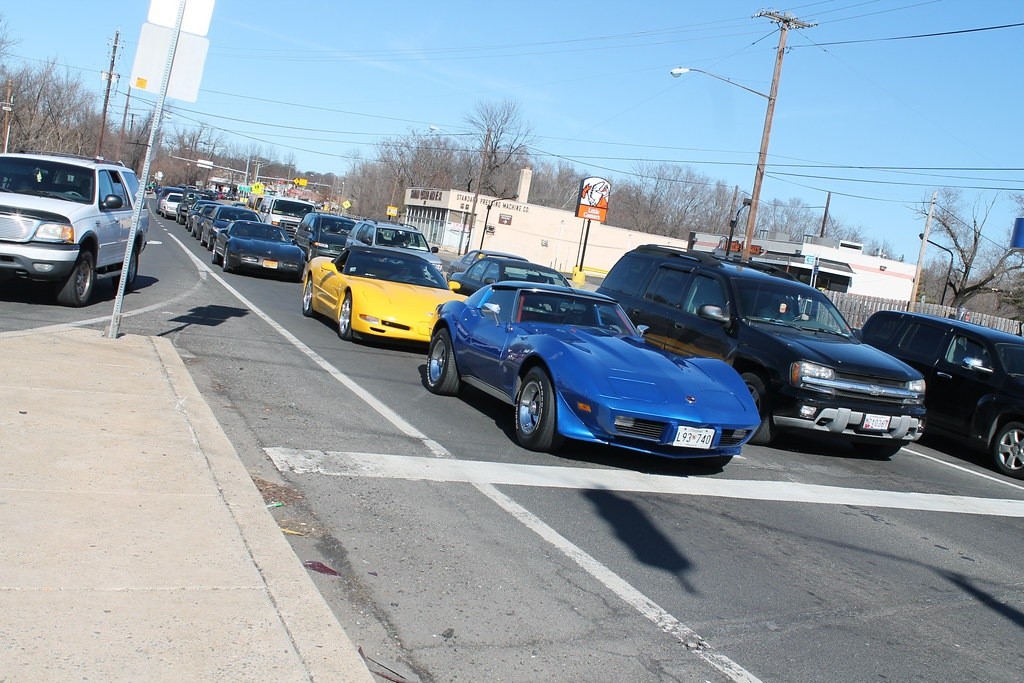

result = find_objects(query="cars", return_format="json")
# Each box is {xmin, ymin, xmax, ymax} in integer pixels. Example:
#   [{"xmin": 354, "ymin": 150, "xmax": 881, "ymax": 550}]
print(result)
[
  {"xmin": 155, "ymin": 184, "xmax": 229, "ymax": 214},
  {"xmin": 446, "ymin": 249, "xmax": 534, "ymax": 284},
  {"xmin": 176, "ymin": 199, "xmax": 196, "ymax": 224},
  {"xmin": 185, "ymin": 199, "xmax": 222, "ymax": 231},
  {"xmin": 159, "ymin": 193, "xmax": 183, "ymax": 219},
  {"xmin": 232, "ymin": 197, "xmax": 264, "ymax": 213},
  {"xmin": 190, "ymin": 204, "xmax": 217, "ymax": 240},
  {"xmin": 201, "ymin": 205, "xmax": 264, "ymax": 251},
  {"xmin": 449, "ymin": 257, "xmax": 588, "ymax": 317}
]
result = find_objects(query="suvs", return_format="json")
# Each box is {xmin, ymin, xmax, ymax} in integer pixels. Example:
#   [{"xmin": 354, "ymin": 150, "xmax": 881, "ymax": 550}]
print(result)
[
  {"xmin": 0, "ymin": 151, "xmax": 150, "ymax": 305},
  {"xmin": 293, "ymin": 212, "xmax": 357, "ymax": 262},
  {"xmin": 343, "ymin": 218, "xmax": 444, "ymax": 278},
  {"xmin": 592, "ymin": 242, "xmax": 926, "ymax": 457}
]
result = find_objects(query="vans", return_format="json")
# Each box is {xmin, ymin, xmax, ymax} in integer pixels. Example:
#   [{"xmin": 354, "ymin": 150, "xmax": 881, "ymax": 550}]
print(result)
[
  {"xmin": 854, "ymin": 309, "xmax": 1023, "ymax": 478},
  {"xmin": 257, "ymin": 195, "xmax": 315, "ymax": 241}
]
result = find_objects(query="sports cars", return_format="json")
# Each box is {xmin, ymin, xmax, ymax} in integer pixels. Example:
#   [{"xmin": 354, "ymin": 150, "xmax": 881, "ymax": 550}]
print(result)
[
  {"xmin": 424, "ymin": 278, "xmax": 762, "ymax": 469},
  {"xmin": 301, "ymin": 244, "xmax": 470, "ymax": 340},
  {"xmin": 212, "ymin": 219, "xmax": 306, "ymax": 281}
]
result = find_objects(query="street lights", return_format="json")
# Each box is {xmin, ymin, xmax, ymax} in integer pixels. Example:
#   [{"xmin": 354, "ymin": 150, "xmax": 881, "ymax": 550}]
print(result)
[
  {"xmin": 671, "ymin": 66, "xmax": 777, "ymax": 262},
  {"xmin": 723, "ymin": 198, "xmax": 752, "ymax": 262},
  {"xmin": 480, "ymin": 194, "xmax": 519, "ymax": 248},
  {"xmin": 428, "ymin": 125, "xmax": 487, "ymax": 254},
  {"xmin": 920, "ymin": 233, "xmax": 954, "ymax": 304}
]
